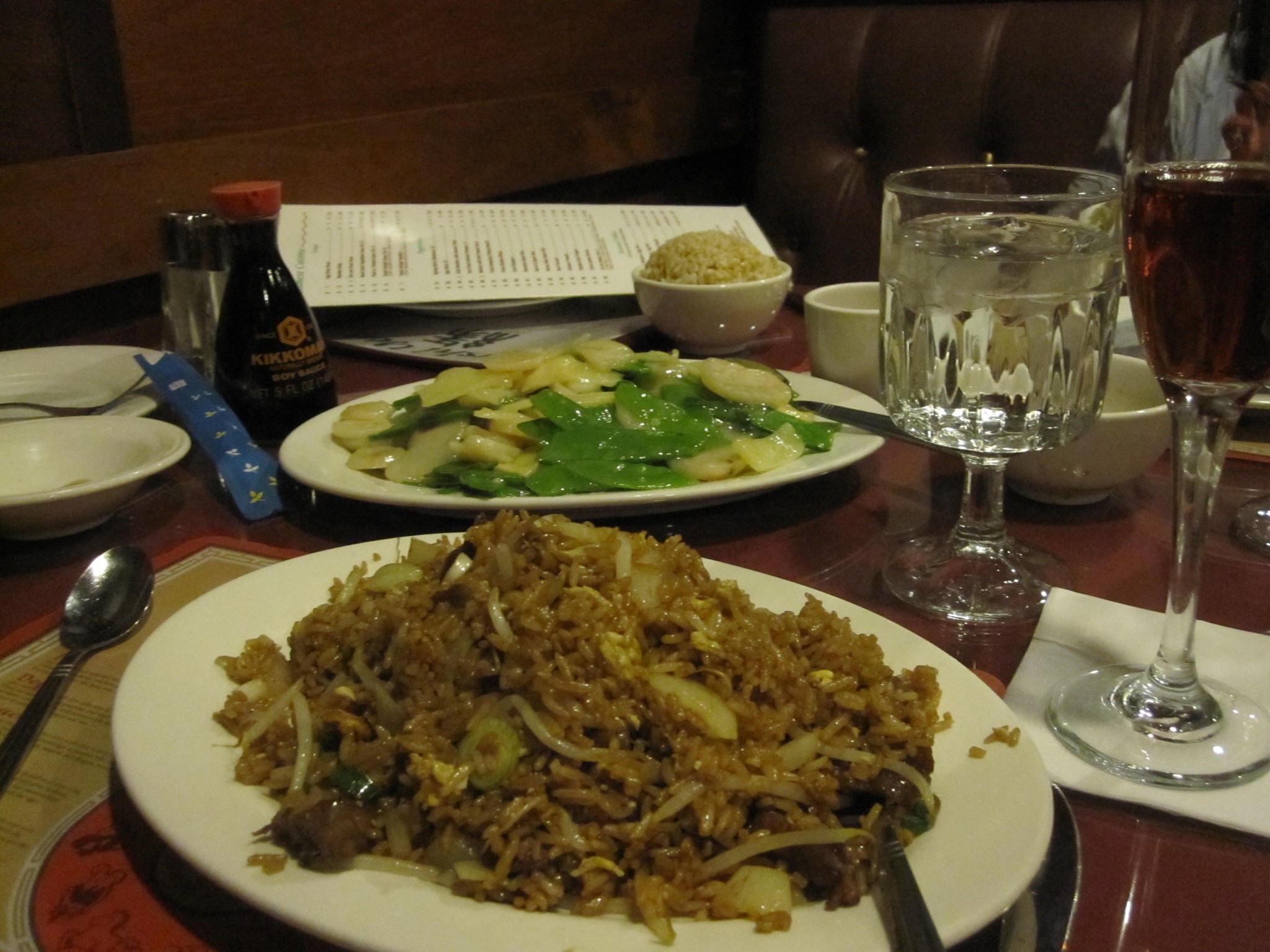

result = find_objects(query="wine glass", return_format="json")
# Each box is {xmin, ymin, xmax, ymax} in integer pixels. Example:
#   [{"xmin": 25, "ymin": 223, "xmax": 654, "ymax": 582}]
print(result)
[
  {"xmin": 871, "ymin": 162, "xmax": 1124, "ymax": 625},
  {"xmin": 1037, "ymin": 0, "xmax": 1270, "ymax": 791}
]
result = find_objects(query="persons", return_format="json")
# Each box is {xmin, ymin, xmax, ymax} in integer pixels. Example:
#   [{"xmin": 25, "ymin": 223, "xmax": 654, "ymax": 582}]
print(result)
[{"xmin": 1168, "ymin": 0, "xmax": 1270, "ymax": 161}]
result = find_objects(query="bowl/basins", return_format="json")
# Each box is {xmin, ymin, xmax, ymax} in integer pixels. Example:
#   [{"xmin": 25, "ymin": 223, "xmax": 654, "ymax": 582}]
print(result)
[
  {"xmin": 1006, "ymin": 352, "xmax": 1176, "ymax": 507},
  {"xmin": 633, "ymin": 257, "xmax": 793, "ymax": 356},
  {"xmin": 0, "ymin": 415, "xmax": 193, "ymax": 542}
]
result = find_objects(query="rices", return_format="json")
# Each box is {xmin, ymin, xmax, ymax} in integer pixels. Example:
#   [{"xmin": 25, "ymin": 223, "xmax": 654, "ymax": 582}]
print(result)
[
  {"xmin": 211, "ymin": 506, "xmax": 1020, "ymax": 935},
  {"xmin": 640, "ymin": 230, "xmax": 781, "ymax": 284}
]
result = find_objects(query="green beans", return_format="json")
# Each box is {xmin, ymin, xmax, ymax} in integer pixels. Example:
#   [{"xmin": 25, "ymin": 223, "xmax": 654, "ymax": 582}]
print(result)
[{"xmin": 368, "ymin": 358, "xmax": 842, "ymax": 499}]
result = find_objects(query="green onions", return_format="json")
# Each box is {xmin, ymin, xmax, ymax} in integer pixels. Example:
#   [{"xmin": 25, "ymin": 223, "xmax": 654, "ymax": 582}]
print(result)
[{"xmin": 323, "ymin": 536, "xmax": 790, "ymax": 943}]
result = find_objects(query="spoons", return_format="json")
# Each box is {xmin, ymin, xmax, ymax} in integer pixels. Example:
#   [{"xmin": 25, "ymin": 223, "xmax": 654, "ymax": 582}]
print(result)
[{"xmin": 0, "ymin": 542, "xmax": 154, "ymax": 791}]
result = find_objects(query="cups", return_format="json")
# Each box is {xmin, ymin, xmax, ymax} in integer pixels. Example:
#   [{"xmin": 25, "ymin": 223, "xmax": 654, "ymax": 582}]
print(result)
[{"xmin": 802, "ymin": 279, "xmax": 883, "ymax": 396}]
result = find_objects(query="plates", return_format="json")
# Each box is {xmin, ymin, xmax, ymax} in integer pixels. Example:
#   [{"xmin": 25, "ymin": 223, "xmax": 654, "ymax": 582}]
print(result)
[
  {"xmin": 103, "ymin": 530, "xmax": 1055, "ymax": 951},
  {"xmin": 0, "ymin": 344, "xmax": 171, "ymax": 422},
  {"xmin": 275, "ymin": 356, "xmax": 890, "ymax": 523},
  {"xmin": 381, "ymin": 297, "xmax": 571, "ymax": 317}
]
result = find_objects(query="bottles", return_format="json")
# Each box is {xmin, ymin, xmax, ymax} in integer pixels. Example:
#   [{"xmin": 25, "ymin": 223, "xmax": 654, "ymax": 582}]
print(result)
[
  {"xmin": 215, "ymin": 179, "xmax": 338, "ymax": 517},
  {"xmin": 157, "ymin": 207, "xmax": 225, "ymax": 387}
]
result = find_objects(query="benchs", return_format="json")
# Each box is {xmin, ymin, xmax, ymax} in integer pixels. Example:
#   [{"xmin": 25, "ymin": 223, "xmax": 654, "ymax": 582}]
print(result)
[{"xmin": 764, "ymin": 0, "xmax": 1240, "ymax": 380}]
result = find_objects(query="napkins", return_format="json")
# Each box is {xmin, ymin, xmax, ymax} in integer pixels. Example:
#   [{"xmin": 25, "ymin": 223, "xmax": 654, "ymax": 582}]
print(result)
[{"xmin": 1004, "ymin": 587, "xmax": 1270, "ymax": 837}]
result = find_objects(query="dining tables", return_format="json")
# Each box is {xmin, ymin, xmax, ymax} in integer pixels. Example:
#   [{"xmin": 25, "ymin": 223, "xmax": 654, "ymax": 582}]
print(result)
[{"xmin": 0, "ymin": 285, "xmax": 1270, "ymax": 952}]
